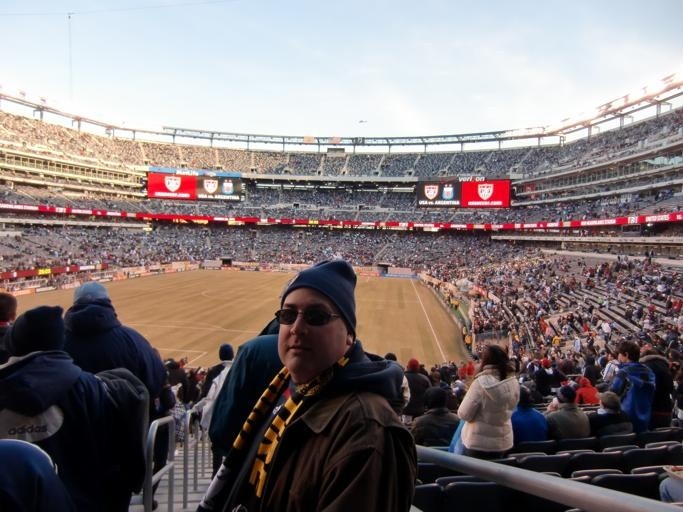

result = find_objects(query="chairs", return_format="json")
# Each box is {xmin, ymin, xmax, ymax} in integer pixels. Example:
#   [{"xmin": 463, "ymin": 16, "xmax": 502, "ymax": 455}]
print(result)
[
  {"xmin": 471, "ymin": 254, "xmax": 682, "ymax": 366},
  {"xmin": 0, "ymin": 438, "xmax": 71, "ymax": 512},
  {"xmin": 412, "ymin": 373, "xmax": 683, "ymax": 512}
]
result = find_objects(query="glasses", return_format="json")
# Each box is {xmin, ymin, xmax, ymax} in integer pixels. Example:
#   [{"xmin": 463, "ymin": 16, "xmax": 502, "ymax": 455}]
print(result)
[{"xmin": 275, "ymin": 308, "xmax": 340, "ymax": 325}]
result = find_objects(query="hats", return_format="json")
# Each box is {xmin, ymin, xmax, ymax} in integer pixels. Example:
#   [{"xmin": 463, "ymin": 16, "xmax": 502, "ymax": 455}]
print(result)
[
  {"xmin": 407, "ymin": 357, "xmax": 420, "ymax": 368},
  {"xmin": 74, "ymin": 281, "xmax": 113, "ymax": 303},
  {"xmin": 281, "ymin": 258, "xmax": 357, "ymax": 341},
  {"xmin": 219, "ymin": 344, "xmax": 233, "ymax": 357},
  {"xmin": 8, "ymin": 306, "xmax": 63, "ymax": 355},
  {"xmin": 557, "ymin": 386, "xmax": 577, "ymax": 402}
]
